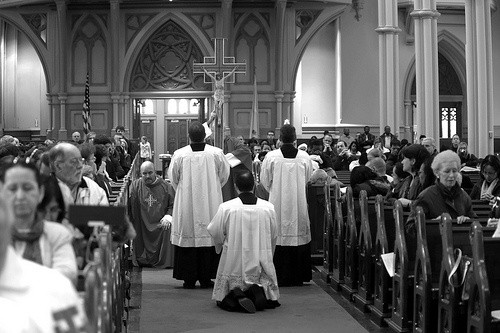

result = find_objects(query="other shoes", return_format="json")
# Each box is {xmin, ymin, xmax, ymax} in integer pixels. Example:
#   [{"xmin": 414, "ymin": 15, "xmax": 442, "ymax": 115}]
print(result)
[
  {"xmin": 183, "ymin": 282, "xmax": 195, "ymax": 289},
  {"xmin": 201, "ymin": 281, "xmax": 214, "ymax": 288},
  {"xmin": 238, "ymin": 298, "xmax": 256, "ymax": 313}
]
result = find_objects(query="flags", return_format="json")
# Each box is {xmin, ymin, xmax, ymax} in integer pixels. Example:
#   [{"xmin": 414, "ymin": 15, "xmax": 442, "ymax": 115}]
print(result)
[
  {"xmin": 81, "ymin": 72, "xmax": 92, "ymax": 140},
  {"xmin": 249, "ymin": 74, "xmax": 259, "ymax": 138}
]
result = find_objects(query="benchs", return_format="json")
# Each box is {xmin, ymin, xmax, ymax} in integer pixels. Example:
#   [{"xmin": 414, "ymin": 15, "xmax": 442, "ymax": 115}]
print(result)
[
  {"xmin": 246, "ymin": 139, "xmax": 500, "ymax": 333},
  {"xmin": 77, "ymin": 151, "xmax": 141, "ymax": 333}
]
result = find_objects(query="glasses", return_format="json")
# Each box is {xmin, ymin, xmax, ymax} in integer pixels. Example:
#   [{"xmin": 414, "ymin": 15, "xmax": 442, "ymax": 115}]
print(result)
[{"xmin": 482, "ymin": 169, "xmax": 496, "ymax": 176}]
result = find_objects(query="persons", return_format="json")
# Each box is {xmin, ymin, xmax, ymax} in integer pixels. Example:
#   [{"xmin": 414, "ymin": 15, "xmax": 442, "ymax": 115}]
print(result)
[
  {"xmin": 167, "ymin": 123, "xmax": 230, "ymax": 288},
  {"xmin": 138, "ymin": 136, "xmax": 152, "ymax": 161},
  {"xmin": 202, "ymin": 65, "xmax": 238, "ymax": 125},
  {"xmin": 207, "ymin": 168, "xmax": 281, "ymax": 313},
  {"xmin": 297, "ymin": 125, "xmax": 500, "ymax": 250},
  {"xmin": 260, "ymin": 124, "xmax": 314, "ymax": 286},
  {"xmin": 237, "ymin": 122, "xmax": 281, "ymax": 167},
  {"xmin": 201, "ymin": 108, "xmax": 252, "ymax": 203},
  {"xmin": 130, "ymin": 161, "xmax": 175, "ymax": 269},
  {"xmin": 0, "ymin": 126, "xmax": 133, "ymax": 333}
]
